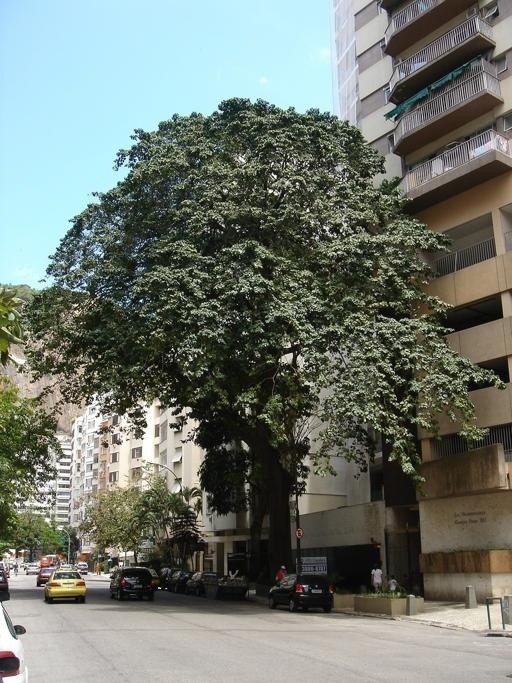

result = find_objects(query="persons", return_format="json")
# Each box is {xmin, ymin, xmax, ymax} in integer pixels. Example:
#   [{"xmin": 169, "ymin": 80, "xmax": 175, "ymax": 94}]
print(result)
[
  {"xmin": 275, "ymin": 564, "xmax": 287, "ymax": 582},
  {"xmin": 95, "ymin": 562, "xmax": 101, "ymax": 575},
  {"xmin": 385, "ymin": 575, "xmax": 400, "ymax": 592},
  {"xmin": 328, "ymin": 584, "xmax": 336, "ymax": 594},
  {"xmin": 370, "ymin": 563, "xmax": 385, "ymax": 593}
]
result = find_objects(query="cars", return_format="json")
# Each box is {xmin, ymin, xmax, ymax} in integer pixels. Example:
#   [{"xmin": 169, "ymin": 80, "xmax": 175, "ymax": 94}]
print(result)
[
  {"xmin": 109, "ymin": 567, "xmax": 248, "ymax": 601},
  {"xmin": 0, "ymin": 600, "xmax": 30, "ymax": 683},
  {"xmin": 0, "ymin": 555, "xmax": 88, "ymax": 604},
  {"xmin": 268, "ymin": 572, "xmax": 334, "ymax": 612}
]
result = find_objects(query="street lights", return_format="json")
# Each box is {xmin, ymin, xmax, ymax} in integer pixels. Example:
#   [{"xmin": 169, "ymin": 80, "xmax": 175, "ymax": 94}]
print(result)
[
  {"xmin": 58, "ymin": 525, "xmax": 70, "ymax": 564},
  {"xmin": 139, "ymin": 457, "xmax": 182, "ymax": 498}
]
